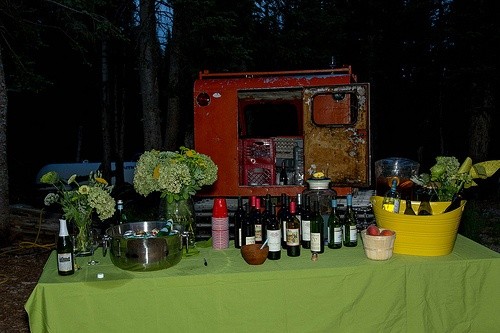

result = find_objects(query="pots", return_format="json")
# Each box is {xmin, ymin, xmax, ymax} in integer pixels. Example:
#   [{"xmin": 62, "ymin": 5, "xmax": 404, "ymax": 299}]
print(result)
[{"xmin": 102, "ymin": 220, "xmax": 189, "ymax": 273}]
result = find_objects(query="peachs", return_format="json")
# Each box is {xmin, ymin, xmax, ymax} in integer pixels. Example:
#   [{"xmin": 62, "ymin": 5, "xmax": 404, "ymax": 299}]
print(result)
[{"xmin": 365, "ymin": 226, "xmax": 395, "ymax": 236}]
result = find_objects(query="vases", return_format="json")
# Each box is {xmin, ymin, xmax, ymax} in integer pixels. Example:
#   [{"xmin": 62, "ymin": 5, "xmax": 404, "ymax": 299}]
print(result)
[{"xmin": 166, "ymin": 193, "xmax": 195, "ymax": 246}]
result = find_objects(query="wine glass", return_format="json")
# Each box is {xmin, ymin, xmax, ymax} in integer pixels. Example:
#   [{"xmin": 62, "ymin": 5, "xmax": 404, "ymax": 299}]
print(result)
[
  {"xmin": 84, "ymin": 230, "xmax": 99, "ymax": 264},
  {"xmin": 69, "ymin": 234, "xmax": 82, "ymax": 270}
]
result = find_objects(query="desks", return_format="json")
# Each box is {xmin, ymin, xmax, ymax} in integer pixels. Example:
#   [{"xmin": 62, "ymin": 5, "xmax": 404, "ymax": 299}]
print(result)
[{"xmin": 24, "ymin": 231, "xmax": 500, "ymax": 332}]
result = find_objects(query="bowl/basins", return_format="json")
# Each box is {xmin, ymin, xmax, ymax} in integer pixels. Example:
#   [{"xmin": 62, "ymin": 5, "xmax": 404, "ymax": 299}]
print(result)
[{"xmin": 241, "ymin": 244, "xmax": 269, "ymax": 265}]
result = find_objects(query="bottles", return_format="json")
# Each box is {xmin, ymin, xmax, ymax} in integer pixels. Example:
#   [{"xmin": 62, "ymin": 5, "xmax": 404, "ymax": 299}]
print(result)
[
  {"xmin": 154, "ymin": 220, "xmax": 173, "ymax": 237},
  {"xmin": 56, "ymin": 215, "xmax": 75, "ymax": 275},
  {"xmin": 444, "ymin": 188, "xmax": 465, "ymax": 213},
  {"xmin": 235, "ymin": 196, "xmax": 246, "ymax": 249},
  {"xmin": 327, "ymin": 196, "xmax": 342, "ymax": 248},
  {"xmin": 113, "ymin": 199, "xmax": 127, "ymax": 226},
  {"xmin": 418, "ymin": 191, "xmax": 433, "ymax": 216},
  {"xmin": 310, "ymin": 202, "xmax": 324, "ymax": 254},
  {"xmin": 383, "ymin": 180, "xmax": 400, "ymax": 214},
  {"xmin": 342, "ymin": 194, "xmax": 358, "ymax": 246},
  {"xmin": 280, "ymin": 162, "xmax": 288, "ymax": 185},
  {"xmin": 403, "ymin": 190, "xmax": 415, "ymax": 214},
  {"xmin": 246, "ymin": 193, "xmax": 311, "ymax": 261}
]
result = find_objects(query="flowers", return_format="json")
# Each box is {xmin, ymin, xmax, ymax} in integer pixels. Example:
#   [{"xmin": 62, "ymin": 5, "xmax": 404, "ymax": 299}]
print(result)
[
  {"xmin": 410, "ymin": 154, "xmax": 500, "ymax": 202},
  {"xmin": 131, "ymin": 146, "xmax": 218, "ymax": 227},
  {"xmin": 39, "ymin": 170, "xmax": 117, "ymax": 249}
]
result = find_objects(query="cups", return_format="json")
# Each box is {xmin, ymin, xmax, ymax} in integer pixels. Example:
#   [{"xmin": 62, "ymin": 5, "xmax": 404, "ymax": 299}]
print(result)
[{"xmin": 211, "ymin": 198, "xmax": 229, "ymax": 249}]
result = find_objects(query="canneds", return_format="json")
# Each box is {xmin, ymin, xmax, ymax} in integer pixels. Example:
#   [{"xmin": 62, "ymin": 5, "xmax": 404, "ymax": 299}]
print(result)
[{"xmin": 124, "ymin": 230, "xmax": 179, "ymax": 239}]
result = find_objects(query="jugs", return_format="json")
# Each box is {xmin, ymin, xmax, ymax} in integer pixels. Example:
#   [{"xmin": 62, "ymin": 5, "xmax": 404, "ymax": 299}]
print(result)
[{"xmin": 374, "ymin": 158, "xmax": 421, "ymax": 188}]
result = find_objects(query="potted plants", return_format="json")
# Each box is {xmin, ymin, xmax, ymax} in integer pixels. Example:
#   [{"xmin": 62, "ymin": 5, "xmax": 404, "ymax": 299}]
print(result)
[{"xmin": 73, "ymin": 219, "xmax": 93, "ymax": 257}]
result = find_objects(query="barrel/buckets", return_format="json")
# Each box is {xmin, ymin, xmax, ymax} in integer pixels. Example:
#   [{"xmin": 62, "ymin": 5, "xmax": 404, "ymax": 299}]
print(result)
[{"xmin": 361, "ymin": 228, "xmax": 395, "ymax": 260}]
